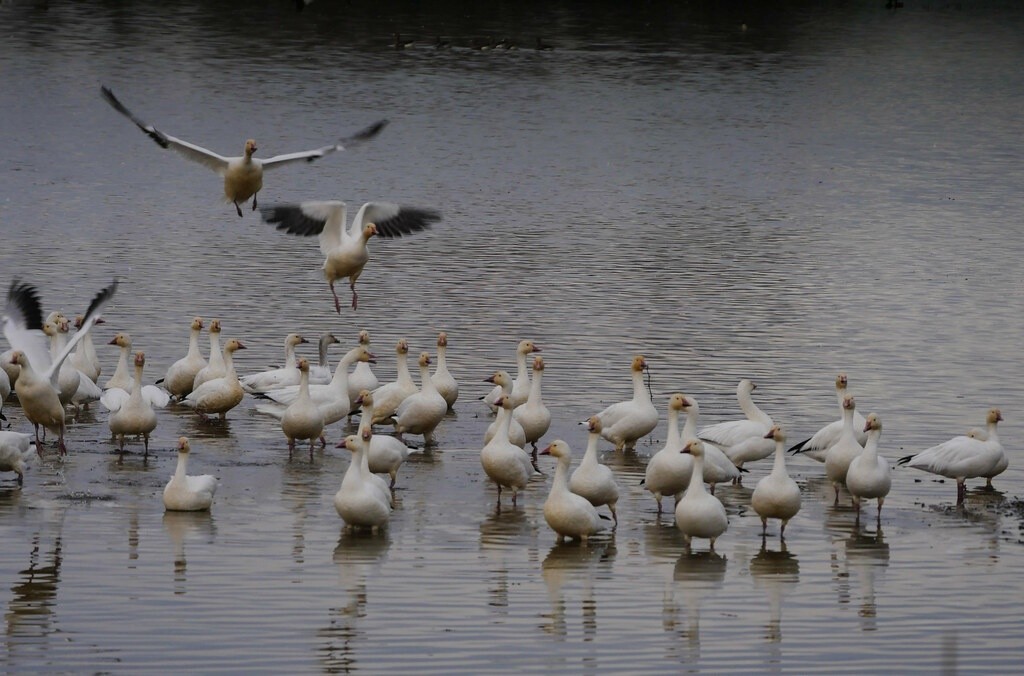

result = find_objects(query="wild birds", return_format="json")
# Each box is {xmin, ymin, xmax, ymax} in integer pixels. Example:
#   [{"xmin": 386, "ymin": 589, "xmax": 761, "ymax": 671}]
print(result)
[
  {"xmin": 161, "ymin": 436, "xmax": 217, "ymax": 511},
  {"xmin": 787, "ymin": 372, "xmax": 869, "ymax": 463},
  {"xmin": 192, "ymin": 320, "xmax": 227, "ymax": 393},
  {"xmin": 0, "ymin": 430, "xmax": 37, "ymax": 488},
  {"xmin": 280, "ymin": 358, "xmax": 324, "ymax": 464},
  {"xmin": 258, "ymin": 198, "xmax": 444, "ymax": 315},
  {"xmin": 1, "ymin": 275, "xmax": 118, "ymax": 460},
  {"xmin": 250, "ymin": 347, "xmax": 378, "ymax": 448},
  {"xmin": 391, "ymin": 351, "xmax": 448, "ymax": 446},
  {"xmin": 155, "ymin": 316, "xmax": 208, "ymax": 402},
  {"xmin": 579, "ymin": 356, "xmax": 660, "ymax": 450},
  {"xmin": 428, "ymin": 331, "xmax": 460, "ymax": 414},
  {"xmin": 333, "ymin": 435, "xmax": 391, "ymax": 525},
  {"xmin": 750, "ymin": 425, "xmax": 801, "ymax": 545},
  {"xmin": 642, "ymin": 394, "xmax": 694, "ymax": 510},
  {"xmin": 360, "ymin": 422, "xmax": 391, "ymax": 504},
  {"xmin": 680, "ymin": 396, "xmax": 740, "ymax": 496},
  {"xmin": 99, "ymin": 85, "xmax": 391, "ymax": 219},
  {"xmin": 846, "ymin": 412, "xmax": 893, "ymax": 522},
  {"xmin": 566, "ymin": 415, "xmax": 620, "ymax": 527},
  {"xmin": 825, "ymin": 393, "xmax": 866, "ymax": 505},
  {"xmin": 347, "ymin": 329, "xmax": 378, "ymax": 423},
  {"xmin": 480, "ymin": 339, "xmax": 544, "ymax": 415},
  {"xmin": 479, "ymin": 394, "xmax": 534, "ymax": 505},
  {"xmin": 482, "ymin": 371, "xmax": 526, "ymax": 450},
  {"xmin": 514, "ymin": 355, "xmax": 551, "ymax": 475},
  {"xmin": 238, "ymin": 333, "xmax": 309, "ymax": 396},
  {"xmin": 176, "ymin": 339, "xmax": 248, "ymax": 423},
  {"xmin": 105, "ymin": 332, "xmax": 134, "ymax": 395},
  {"xmin": 348, "ymin": 339, "xmax": 418, "ymax": 432},
  {"xmin": 897, "ymin": 428, "xmax": 1002, "ymax": 505},
  {"xmin": 674, "ymin": 440, "xmax": 728, "ymax": 550},
  {"xmin": 983, "ymin": 408, "xmax": 1009, "ymax": 484},
  {"xmin": 696, "ymin": 379, "xmax": 777, "ymax": 486},
  {"xmin": 99, "ymin": 350, "xmax": 172, "ymax": 452},
  {"xmin": 537, "ymin": 440, "xmax": 604, "ymax": 543},
  {"xmin": 353, "ymin": 389, "xmax": 409, "ymax": 489},
  {"xmin": 299, "ymin": 335, "xmax": 340, "ymax": 385}
]
